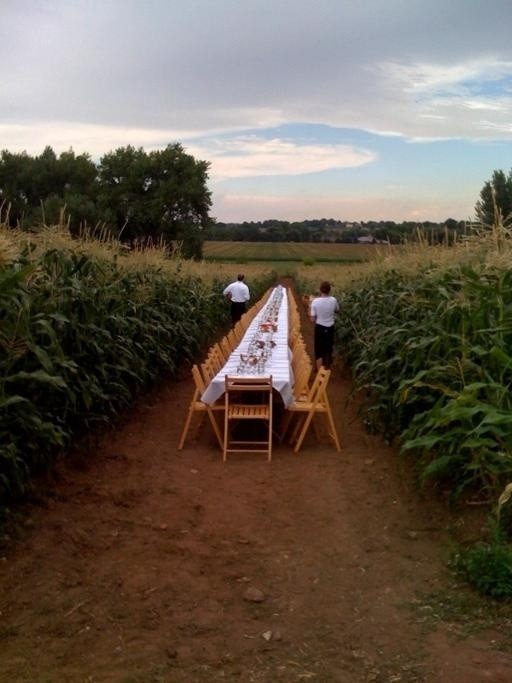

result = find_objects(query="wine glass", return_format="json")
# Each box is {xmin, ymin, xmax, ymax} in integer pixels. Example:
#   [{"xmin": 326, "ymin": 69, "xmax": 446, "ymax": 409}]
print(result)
[{"xmin": 238, "ymin": 283, "xmax": 284, "ymax": 373}]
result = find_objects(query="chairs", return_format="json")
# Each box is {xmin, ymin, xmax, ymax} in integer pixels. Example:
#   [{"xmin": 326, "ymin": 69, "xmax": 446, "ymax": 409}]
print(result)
[{"xmin": 176, "ymin": 284, "xmax": 342, "ymax": 462}]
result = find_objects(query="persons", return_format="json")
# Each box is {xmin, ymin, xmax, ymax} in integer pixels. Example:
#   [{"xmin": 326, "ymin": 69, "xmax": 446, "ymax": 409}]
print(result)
[
  {"xmin": 307, "ymin": 280, "xmax": 339, "ymax": 372},
  {"xmin": 222, "ymin": 272, "xmax": 251, "ymax": 328}
]
